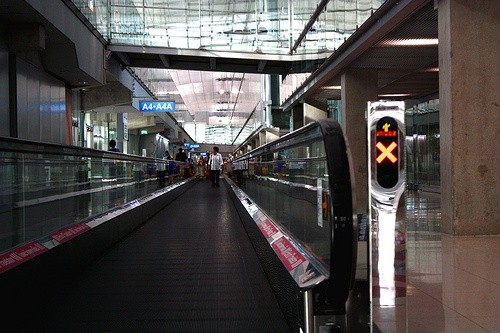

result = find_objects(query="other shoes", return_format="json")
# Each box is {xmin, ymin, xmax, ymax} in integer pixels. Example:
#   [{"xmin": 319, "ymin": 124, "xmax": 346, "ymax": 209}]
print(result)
[{"xmin": 211, "ymin": 183, "xmax": 220, "ymax": 188}]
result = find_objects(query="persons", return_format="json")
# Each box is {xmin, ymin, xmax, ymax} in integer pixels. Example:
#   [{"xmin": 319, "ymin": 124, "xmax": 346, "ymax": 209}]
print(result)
[
  {"xmin": 155, "ymin": 142, "xmax": 166, "ymax": 190},
  {"xmin": 232, "ymin": 161, "xmax": 245, "ymax": 188},
  {"xmin": 163, "ymin": 148, "xmax": 195, "ymax": 186},
  {"xmin": 204, "ymin": 153, "xmax": 212, "ymax": 173},
  {"xmin": 102, "ymin": 140, "xmax": 124, "ymax": 209},
  {"xmin": 209, "ymin": 146, "xmax": 223, "ymax": 188},
  {"xmin": 197, "ymin": 156, "xmax": 206, "ymax": 179}
]
